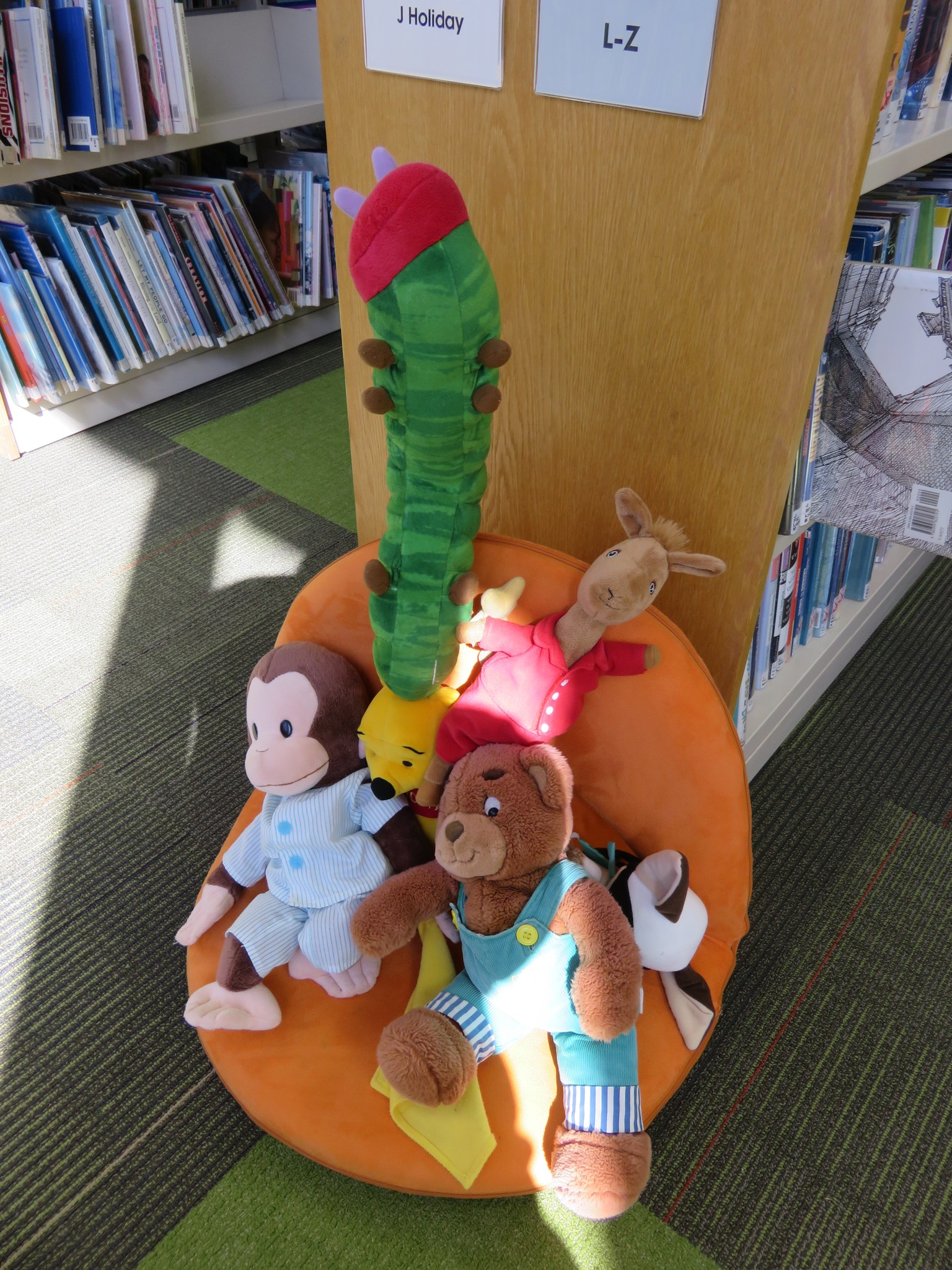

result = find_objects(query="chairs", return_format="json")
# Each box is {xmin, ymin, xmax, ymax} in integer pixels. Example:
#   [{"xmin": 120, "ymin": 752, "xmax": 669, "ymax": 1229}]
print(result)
[{"xmin": 170, "ymin": 523, "xmax": 761, "ymax": 1213}]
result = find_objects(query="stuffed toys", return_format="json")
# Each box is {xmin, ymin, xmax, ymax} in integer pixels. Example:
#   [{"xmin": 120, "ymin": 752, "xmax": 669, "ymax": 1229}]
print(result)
[{"xmin": 176, "ymin": 163, "xmax": 726, "ymax": 1220}]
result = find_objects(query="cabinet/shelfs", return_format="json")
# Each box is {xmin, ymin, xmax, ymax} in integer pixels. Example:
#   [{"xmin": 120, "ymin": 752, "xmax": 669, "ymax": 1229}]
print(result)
[
  {"xmin": 0, "ymin": 0, "xmax": 340, "ymax": 462},
  {"xmin": 315, "ymin": 0, "xmax": 952, "ymax": 780}
]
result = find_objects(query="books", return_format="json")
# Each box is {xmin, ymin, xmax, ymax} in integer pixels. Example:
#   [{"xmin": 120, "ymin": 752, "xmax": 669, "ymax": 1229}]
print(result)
[
  {"xmin": 738, "ymin": 0, "xmax": 952, "ymax": 730},
  {"xmin": 0, "ymin": 0, "xmax": 341, "ymax": 415}
]
